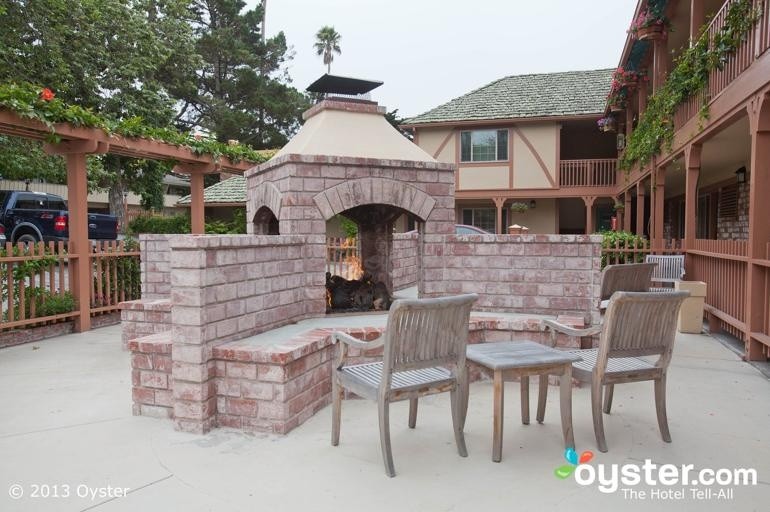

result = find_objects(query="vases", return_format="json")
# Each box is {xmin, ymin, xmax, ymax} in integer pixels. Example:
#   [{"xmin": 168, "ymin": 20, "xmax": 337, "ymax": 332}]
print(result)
[{"xmin": 637, "ymin": 23, "xmax": 664, "ymax": 41}]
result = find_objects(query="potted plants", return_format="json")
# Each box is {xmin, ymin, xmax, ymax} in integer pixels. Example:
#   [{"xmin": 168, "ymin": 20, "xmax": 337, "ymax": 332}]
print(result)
[{"xmin": 613, "ymin": 201, "xmax": 625, "ymax": 214}]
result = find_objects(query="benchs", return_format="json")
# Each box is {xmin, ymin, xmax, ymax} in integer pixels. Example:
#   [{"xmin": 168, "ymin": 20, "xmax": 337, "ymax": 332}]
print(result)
[
  {"xmin": 116, "ymin": 233, "xmax": 175, "ymax": 423},
  {"xmin": 172, "ymin": 232, "xmax": 604, "ymax": 437}
]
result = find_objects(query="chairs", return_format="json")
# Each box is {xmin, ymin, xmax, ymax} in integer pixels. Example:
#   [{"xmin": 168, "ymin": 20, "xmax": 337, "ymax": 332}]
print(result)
[
  {"xmin": 597, "ymin": 262, "xmax": 661, "ymax": 314},
  {"xmin": 539, "ymin": 289, "xmax": 697, "ymax": 455},
  {"xmin": 645, "ymin": 254, "xmax": 687, "ymax": 294},
  {"xmin": 329, "ymin": 292, "xmax": 482, "ymax": 479}
]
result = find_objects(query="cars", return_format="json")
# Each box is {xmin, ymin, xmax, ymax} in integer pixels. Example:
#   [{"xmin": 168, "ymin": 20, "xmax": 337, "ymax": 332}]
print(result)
[{"xmin": 405, "ymin": 223, "xmax": 490, "ymax": 235}]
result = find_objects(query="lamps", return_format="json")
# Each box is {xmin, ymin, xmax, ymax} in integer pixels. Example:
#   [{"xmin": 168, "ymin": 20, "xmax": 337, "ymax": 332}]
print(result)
[
  {"xmin": 529, "ymin": 199, "xmax": 537, "ymax": 209},
  {"xmin": 735, "ymin": 165, "xmax": 748, "ymax": 184}
]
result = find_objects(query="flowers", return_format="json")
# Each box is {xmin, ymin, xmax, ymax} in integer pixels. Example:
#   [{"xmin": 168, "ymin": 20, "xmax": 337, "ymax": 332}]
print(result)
[
  {"xmin": 625, "ymin": 6, "xmax": 676, "ymax": 40},
  {"xmin": 596, "ymin": 64, "xmax": 649, "ymax": 134}
]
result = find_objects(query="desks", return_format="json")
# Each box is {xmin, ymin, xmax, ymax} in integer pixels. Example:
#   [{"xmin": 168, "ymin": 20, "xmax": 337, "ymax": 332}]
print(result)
[{"xmin": 464, "ymin": 338, "xmax": 581, "ymax": 463}]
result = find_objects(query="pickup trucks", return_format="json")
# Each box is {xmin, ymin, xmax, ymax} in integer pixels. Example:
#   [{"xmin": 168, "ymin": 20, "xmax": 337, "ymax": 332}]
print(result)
[{"xmin": 0, "ymin": 190, "xmax": 119, "ymax": 262}]
